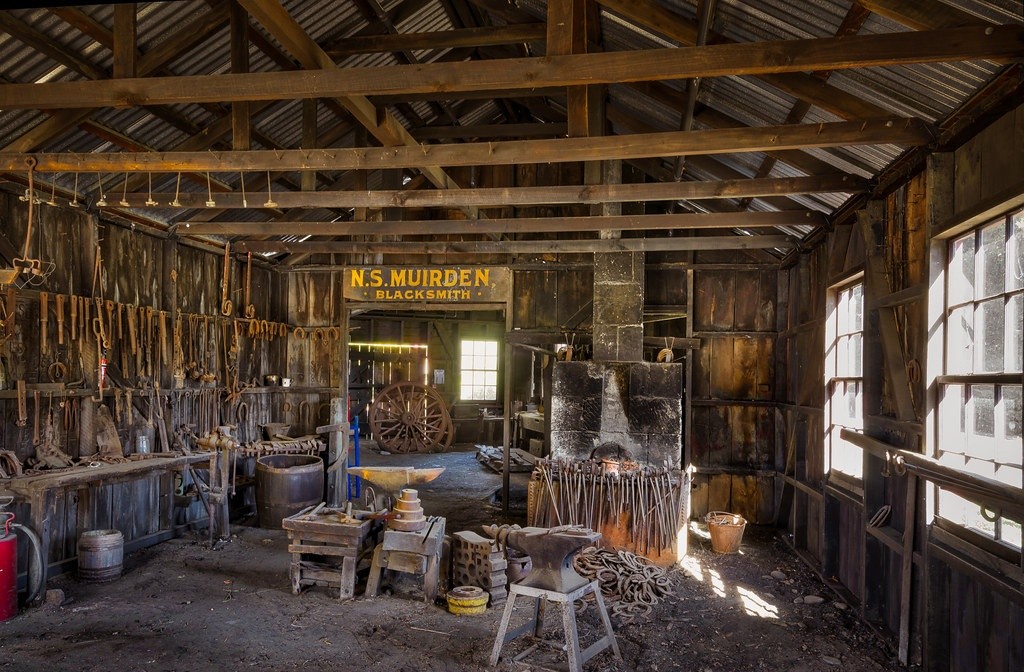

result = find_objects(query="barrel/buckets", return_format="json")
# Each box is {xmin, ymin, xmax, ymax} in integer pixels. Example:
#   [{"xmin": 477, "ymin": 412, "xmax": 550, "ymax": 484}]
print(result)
[
  {"xmin": 706, "ymin": 510, "xmax": 748, "ymax": 554},
  {"xmin": 254, "ymin": 454, "xmax": 324, "ymax": 530},
  {"xmin": 78, "ymin": 529, "xmax": 124, "ymax": 583}
]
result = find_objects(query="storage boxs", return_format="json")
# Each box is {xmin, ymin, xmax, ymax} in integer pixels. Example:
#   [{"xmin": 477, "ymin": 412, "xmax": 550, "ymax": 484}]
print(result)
[
  {"xmin": 530, "ymin": 439, "xmax": 545, "ymax": 458},
  {"xmin": 454, "ymin": 404, "xmax": 479, "ymax": 419}
]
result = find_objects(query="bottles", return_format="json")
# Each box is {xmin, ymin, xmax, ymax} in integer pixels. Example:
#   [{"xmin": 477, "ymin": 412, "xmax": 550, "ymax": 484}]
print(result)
[
  {"xmin": 136, "ymin": 435, "xmax": 150, "ymax": 455},
  {"xmin": 98, "ymin": 359, "xmax": 107, "ymax": 388}
]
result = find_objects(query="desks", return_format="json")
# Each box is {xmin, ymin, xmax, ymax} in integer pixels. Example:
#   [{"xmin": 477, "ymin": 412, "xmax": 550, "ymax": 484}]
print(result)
[
  {"xmin": 0, "ymin": 450, "xmax": 218, "ymax": 609},
  {"xmin": 513, "ymin": 412, "xmax": 544, "ymax": 448},
  {"xmin": 452, "ymin": 417, "xmax": 513, "ymax": 447}
]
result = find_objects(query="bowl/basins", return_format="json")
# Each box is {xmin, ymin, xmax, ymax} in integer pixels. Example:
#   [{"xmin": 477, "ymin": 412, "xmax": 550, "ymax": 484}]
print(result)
[{"xmin": 259, "ymin": 422, "xmax": 291, "ymax": 442}]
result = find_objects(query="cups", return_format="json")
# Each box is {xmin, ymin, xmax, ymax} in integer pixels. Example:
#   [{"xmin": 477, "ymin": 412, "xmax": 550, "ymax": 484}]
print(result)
[
  {"xmin": 282, "ymin": 377, "xmax": 290, "ymax": 387},
  {"xmin": 264, "ymin": 374, "xmax": 279, "ymax": 386}
]
